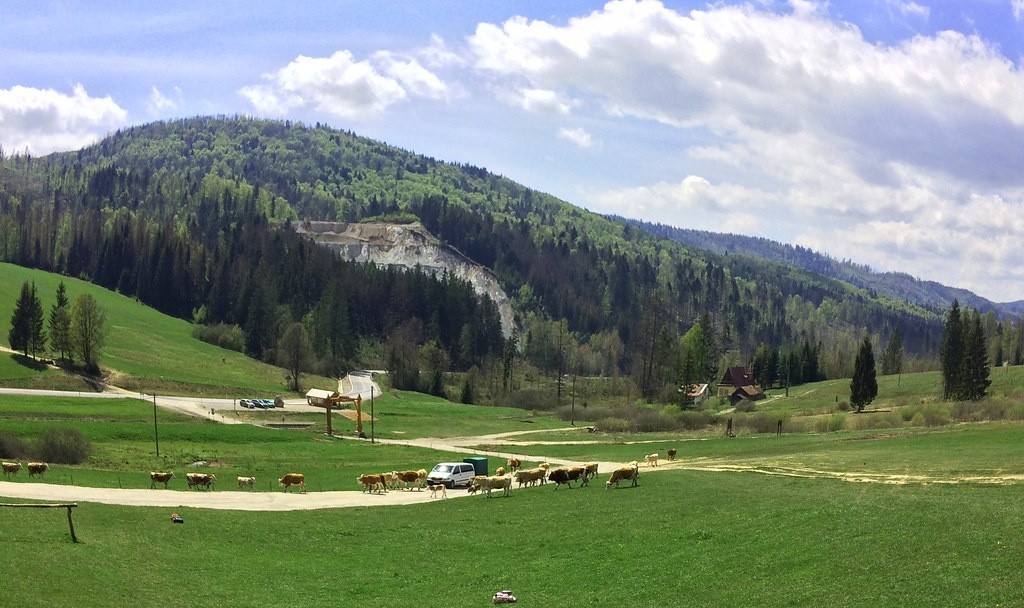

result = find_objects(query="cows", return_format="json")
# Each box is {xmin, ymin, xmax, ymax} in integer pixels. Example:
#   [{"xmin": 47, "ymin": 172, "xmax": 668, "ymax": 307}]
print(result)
[
  {"xmin": 606, "ymin": 461, "xmax": 639, "ymax": 489},
  {"xmin": 186, "ymin": 472, "xmax": 215, "ymax": 490},
  {"xmin": 1, "ymin": 462, "xmax": 23, "ymax": 477},
  {"xmin": 357, "ymin": 469, "xmax": 427, "ymax": 494},
  {"xmin": 668, "ymin": 448, "xmax": 677, "ymax": 461},
  {"xmin": 427, "ymin": 484, "xmax": 447, "ymax": 498},
  {"xmin": 150, "ymin": 471, "xmax": 176, "ymax": 489},
  {"xmin": 644, "ymin": 453, "xmax": 658, "ymax": 467},
  {"xmin": 466, "ymin": 459, "xmax": 550, "ymax": 497},
  {"xmin": 278, "ymin": 474, "xmax": 306, "ymax": 493},
  {"xmin": 548, "ymin": 463, "xmax": 598, "ymax": 490},
  {"xmin": 237, "ymin": 477, "xmax": 256, "ymax": 490},
  {"xmin": 27, "ymin": 463, "xmax": 50, "ymax": 479}
]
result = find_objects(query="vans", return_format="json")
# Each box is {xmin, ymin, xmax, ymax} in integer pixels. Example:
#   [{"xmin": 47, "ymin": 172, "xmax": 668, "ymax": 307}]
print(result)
[{"xmin": 427, "ymin": 462, "xmax": 475, "ymax": 489}]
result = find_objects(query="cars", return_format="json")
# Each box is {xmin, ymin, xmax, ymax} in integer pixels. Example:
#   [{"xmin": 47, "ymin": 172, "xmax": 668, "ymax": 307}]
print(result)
[
  {"xmin": 240, "ymin": 399, "xmax": 255, "ymax": 409},
  {"xmin": 262, "ymin": 399, "xmax": 276, "ymax": 408},
  {"xmin": 252, "ymin": 399, "xmax": 269, "ymax": 409}
]
